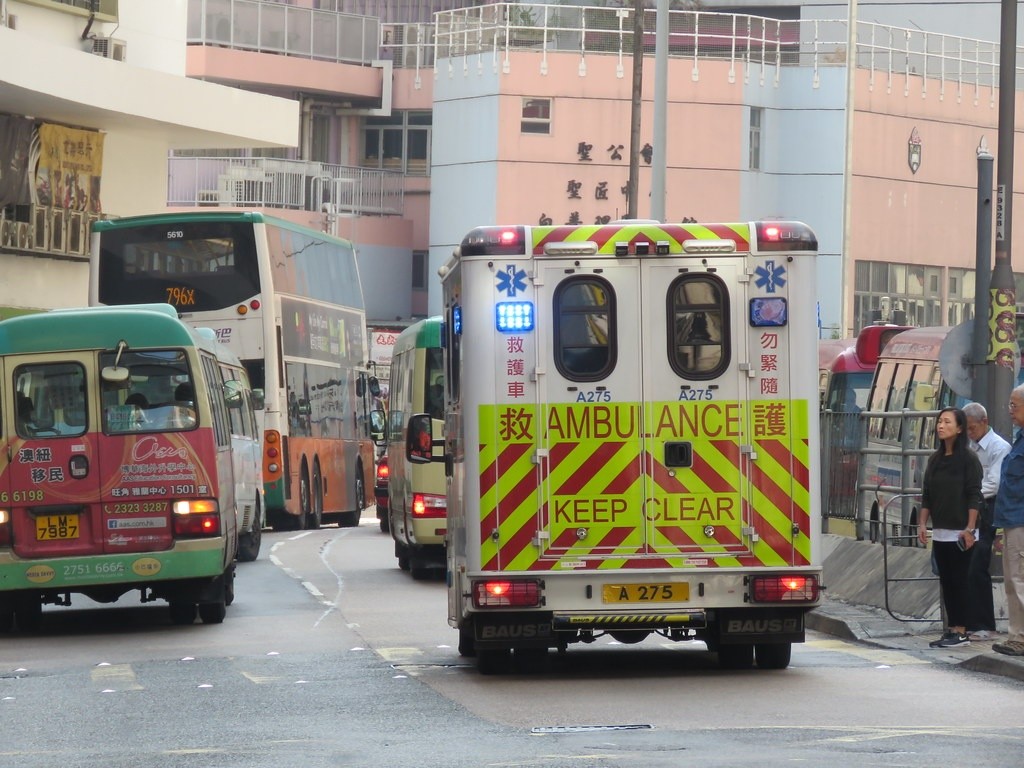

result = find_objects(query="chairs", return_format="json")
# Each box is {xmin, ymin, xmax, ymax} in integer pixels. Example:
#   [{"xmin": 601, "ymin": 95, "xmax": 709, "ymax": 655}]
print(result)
[
  {"xmin": 125, "ymin": 393, "xmax": 150, "ymax": 426},
  {"xmin": 134, "ymin": 271, "xmax": 163, "ymax": 277},
  {"xmin": 174, "ymin": 383, "xmax": 196, "ymax": 412},
  {"xmin": 215, "ymin": 264, "xmax": 236, "ymax": 275}
]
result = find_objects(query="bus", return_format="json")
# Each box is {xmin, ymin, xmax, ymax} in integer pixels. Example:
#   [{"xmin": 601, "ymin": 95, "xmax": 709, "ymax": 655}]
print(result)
[
  {"xmin": 384, "ymin": 316, "xmax": 459, "ymax": 580},
  {"xmin": 857, "ymin": 326, "xmax": 977, "ymax": 547},
  {"xmin": 824, "ymin": 324, "xmax": 919, "ymax": 538},
  {"xmin": 89, "ymin": 212, "xmax": 381, "ymax": 531},
  {"xmin": 0, "ymin": 302, "xmax": 264, "ymax": 628}
]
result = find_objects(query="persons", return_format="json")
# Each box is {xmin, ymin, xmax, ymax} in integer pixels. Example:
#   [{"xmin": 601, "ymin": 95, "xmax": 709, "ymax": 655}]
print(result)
[
  {"xmin": 176, "ymin": 382, "xmax": 193, "ymax": 401},
  {"xmin": 125, "ymin": 393, "xmax": 148, "ymax": 409},
  {"xmin": 919, "ymin": 382, "xmax": 1024, "ymax": 656}
]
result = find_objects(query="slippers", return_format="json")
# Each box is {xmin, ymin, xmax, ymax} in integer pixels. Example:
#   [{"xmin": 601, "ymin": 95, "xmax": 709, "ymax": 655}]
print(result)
[{"xmin": 966, "ymin": 630, "xmax": 998, "ymax": 640}]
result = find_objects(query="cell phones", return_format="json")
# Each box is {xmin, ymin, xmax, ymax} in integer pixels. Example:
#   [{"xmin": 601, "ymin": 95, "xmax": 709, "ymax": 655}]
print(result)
[{"xmin": 958, "ymin": 537, "xmax": 967, "ymax": 551}]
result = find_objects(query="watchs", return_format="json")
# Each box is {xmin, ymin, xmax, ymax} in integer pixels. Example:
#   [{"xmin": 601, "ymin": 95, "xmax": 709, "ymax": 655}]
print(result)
[{"xmin": 965, "ymin": 527, "xmax": 976, "ymax": 534}]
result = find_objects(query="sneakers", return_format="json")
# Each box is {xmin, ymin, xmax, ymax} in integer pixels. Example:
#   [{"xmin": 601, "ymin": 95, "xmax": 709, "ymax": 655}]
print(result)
[{"xmin": 930, "ymin": 632, "xmax": 970, "ymax": 647}]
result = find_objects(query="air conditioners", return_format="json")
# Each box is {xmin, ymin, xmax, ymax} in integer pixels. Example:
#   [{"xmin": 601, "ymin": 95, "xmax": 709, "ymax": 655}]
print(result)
[{"xmin": 94, "ymin": 37, "xmax": 128, "ymax": 62}]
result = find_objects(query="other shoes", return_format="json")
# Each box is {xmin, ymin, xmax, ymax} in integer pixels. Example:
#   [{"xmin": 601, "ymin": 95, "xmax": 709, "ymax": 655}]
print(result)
[{"xmin": 992, "ymin": 640, "xmax": 1024, "ymax": 656}]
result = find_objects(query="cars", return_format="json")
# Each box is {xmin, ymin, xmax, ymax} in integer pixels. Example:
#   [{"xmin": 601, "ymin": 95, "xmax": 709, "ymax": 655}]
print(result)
[{"xmin": 374, "ymin": 446, "xmax": 392, "ymax": 531}]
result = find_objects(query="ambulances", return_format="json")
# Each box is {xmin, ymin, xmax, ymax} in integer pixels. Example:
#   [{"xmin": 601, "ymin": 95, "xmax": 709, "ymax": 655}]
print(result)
[{"xmin": 441, "ymin": 220, "xmax": 822, "ymax": 675}]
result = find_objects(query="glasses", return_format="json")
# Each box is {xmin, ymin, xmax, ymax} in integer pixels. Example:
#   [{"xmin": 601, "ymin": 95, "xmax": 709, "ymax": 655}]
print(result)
[{"xmin": 1007, "ymin": 403, "xmax": 1023, "ymax": 411}]
